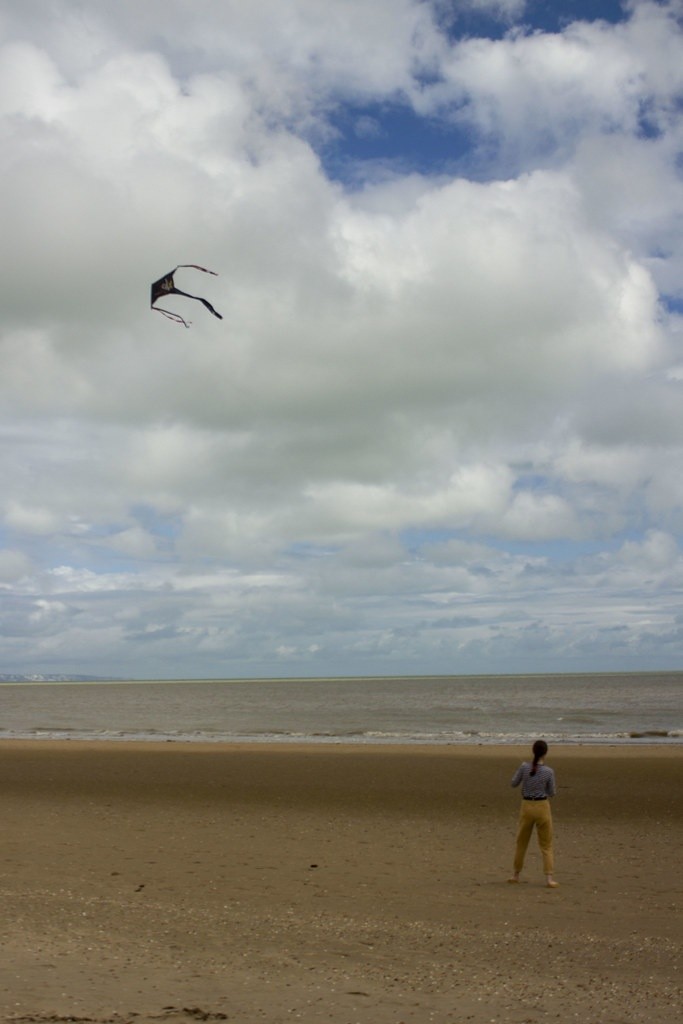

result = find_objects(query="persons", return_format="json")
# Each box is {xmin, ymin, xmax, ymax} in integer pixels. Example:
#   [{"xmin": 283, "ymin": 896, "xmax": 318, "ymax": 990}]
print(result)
[{"xmin": 510, "ymin": 740, "xmax": 557, "ymax": 886}]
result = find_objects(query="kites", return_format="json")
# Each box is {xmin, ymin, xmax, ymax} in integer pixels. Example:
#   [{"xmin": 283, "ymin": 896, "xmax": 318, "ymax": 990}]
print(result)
[{"xmin": 150, "ymin": 264, "xmax": 222, "ymax": 329}]
